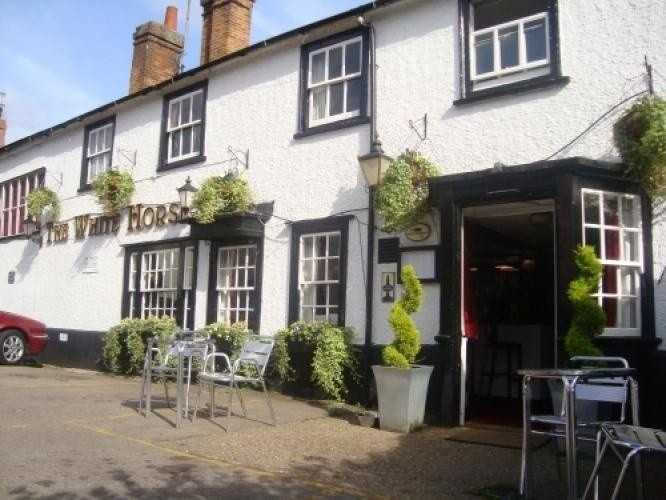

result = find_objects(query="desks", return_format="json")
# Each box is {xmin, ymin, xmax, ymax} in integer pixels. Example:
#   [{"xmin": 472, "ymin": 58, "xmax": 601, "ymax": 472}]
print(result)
[{"xmin": 516, "ymin": 368, "xmax": 643, "ymax": 500}]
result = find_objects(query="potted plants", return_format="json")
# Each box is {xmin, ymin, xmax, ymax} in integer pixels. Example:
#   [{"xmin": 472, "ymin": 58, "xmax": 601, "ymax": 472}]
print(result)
[
  {"xmin": 274, "ymin": 320, "xmax": 364, "ymax": 403},
  {"xmin": 546, "ymin": 242, "xmax": 607, "ymax": 458},
  {"xmin": 369, "ymin": 263, "xmax": 435, "ymax": 434}
]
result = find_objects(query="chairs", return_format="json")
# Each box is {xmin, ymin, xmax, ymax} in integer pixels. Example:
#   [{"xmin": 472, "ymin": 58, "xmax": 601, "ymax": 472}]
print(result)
[
  {"xmin": 138, "ymin": 332, "xmax": 275, "ymax": 430},
  {"xmin": 581, "ymin": 421, "xmax": 666, "ymax": 500},
  {"xmin": 532, "ymin": 355, "xmax": 630, "ymax": 500}
]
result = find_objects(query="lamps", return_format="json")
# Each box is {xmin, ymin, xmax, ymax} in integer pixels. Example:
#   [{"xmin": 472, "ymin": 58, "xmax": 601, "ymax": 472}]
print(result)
[
  {"xmin": 176, "ymin": 175, "xmax": 200, "ymax": 213},
  {"xmin": 357, "ymin": 129, "xmax": 394, "ymax": 189},
  {"xmin": 23, "ymin": 215, "xmax": 42, "ymax": 247}
]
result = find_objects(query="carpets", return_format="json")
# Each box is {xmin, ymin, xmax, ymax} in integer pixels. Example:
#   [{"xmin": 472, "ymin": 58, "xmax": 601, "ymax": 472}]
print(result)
[{"xmin": 443, "ymin": 426, "xmax": 555, "ymax": 451}]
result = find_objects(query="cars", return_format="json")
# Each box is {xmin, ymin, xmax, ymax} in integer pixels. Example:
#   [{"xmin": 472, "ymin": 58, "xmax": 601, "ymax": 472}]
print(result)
[{"xmin": 0, "ymin": 309, "xmax": 50, "ymax": 365}]
